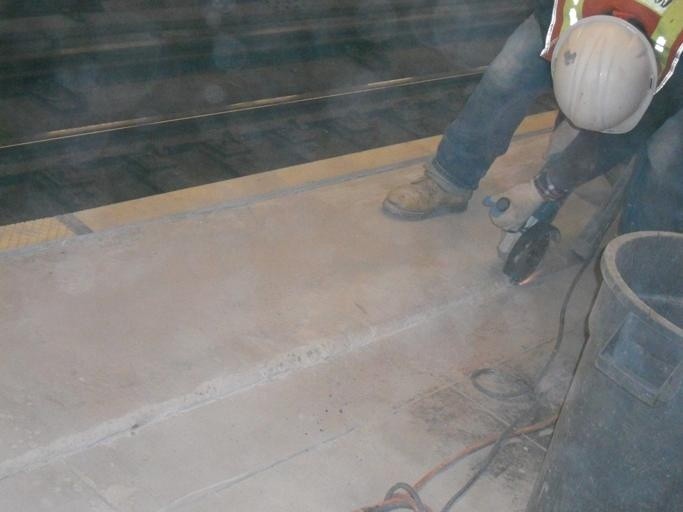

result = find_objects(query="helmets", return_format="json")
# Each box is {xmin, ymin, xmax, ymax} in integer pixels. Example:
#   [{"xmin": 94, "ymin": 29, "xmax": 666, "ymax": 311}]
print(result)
[{"xmin": 550, "ymin": 14, "xmax": 658, "ymax": 135}]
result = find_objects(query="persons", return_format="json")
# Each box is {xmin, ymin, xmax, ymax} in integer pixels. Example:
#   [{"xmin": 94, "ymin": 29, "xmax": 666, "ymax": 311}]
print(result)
[{"xmin": 382, "ymin": 0, "xmax": 682, "ymax": 231}]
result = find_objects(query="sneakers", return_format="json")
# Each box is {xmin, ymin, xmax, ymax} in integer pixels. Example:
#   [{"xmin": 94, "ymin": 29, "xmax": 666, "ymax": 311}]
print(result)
[{"xmin": 382, "ymin": 176, "xmax": 468, "ymax": 220}]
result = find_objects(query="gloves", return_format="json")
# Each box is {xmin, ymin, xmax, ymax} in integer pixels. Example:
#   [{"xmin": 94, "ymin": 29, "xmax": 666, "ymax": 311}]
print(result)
[{"xmin": 483, "ymin": 180, "xmax": 545, "ymax": 233}]
